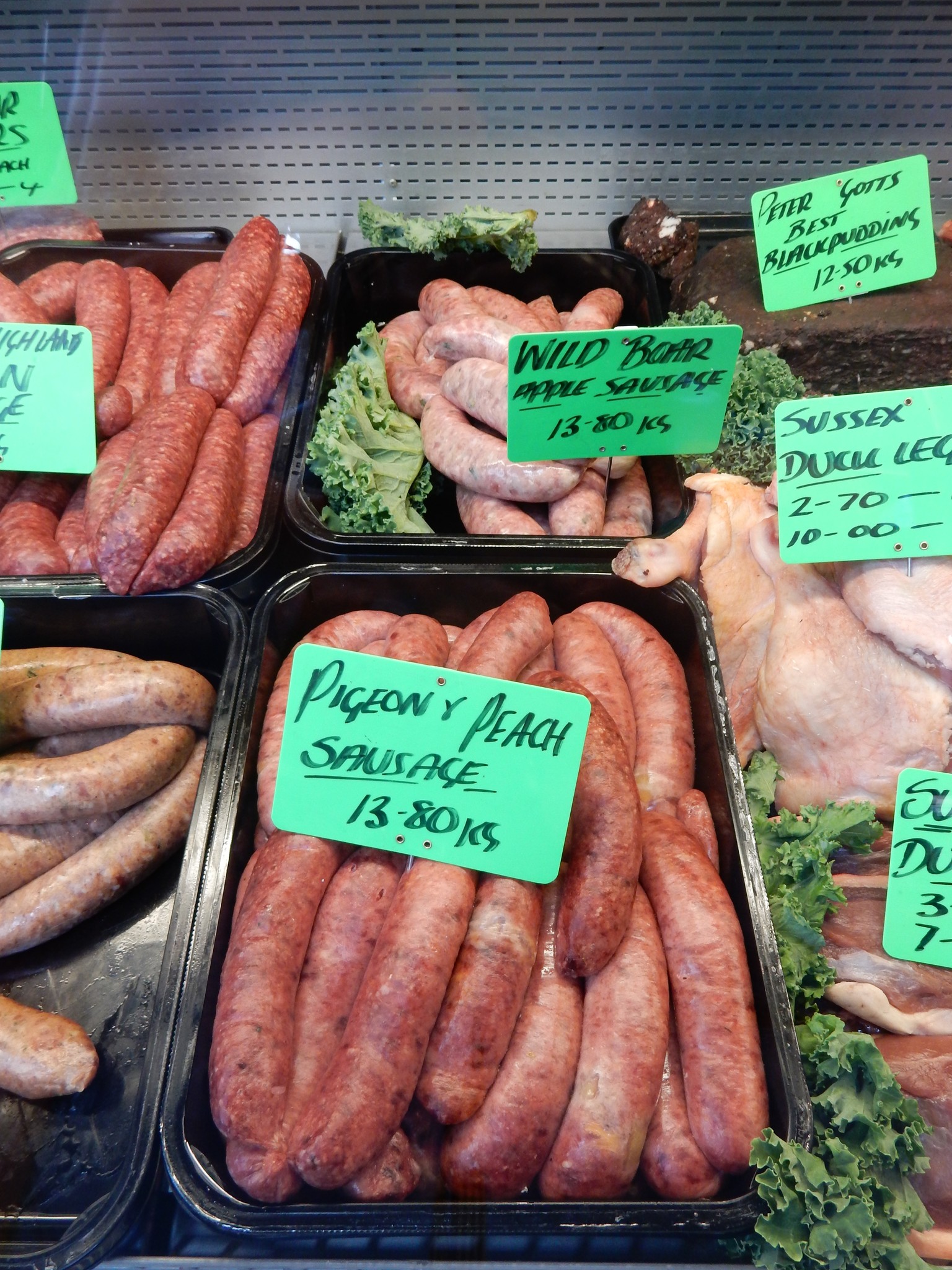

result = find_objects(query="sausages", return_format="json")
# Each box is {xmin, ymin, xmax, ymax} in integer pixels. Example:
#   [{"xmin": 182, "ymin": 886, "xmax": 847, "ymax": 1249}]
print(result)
[{"xmin": 0, "ymin": 215, "xmax": 772, "ymax": 1211}]
